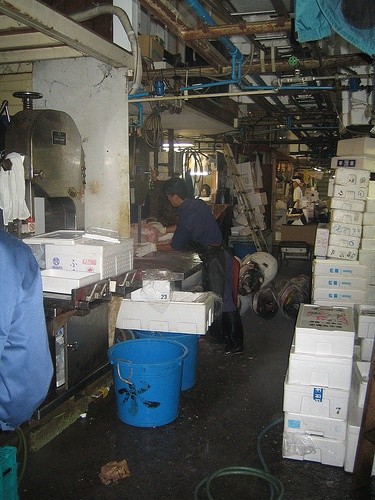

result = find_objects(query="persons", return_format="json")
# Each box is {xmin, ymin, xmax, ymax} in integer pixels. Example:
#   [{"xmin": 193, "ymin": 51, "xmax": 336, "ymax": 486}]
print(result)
[
  {"xmin": 0, "ymin": 225, "xmax": 55, "ymax": 446},
  {"xmin": 136, "ymin": 177, "xmax": 245, "ymax": 357},
  {"xmin": 200, "ymin": 183, "xmax": 210, "ymax": 197},
  {"xmin": 287, "ymin": 178, "xmax": 308, "ymax": 225}
]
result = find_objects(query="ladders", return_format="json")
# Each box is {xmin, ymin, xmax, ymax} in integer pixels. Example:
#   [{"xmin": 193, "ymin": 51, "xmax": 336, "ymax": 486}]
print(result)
[{"xmin": 220, "ymin": 143, "xmax": 267, "ymax": 254}]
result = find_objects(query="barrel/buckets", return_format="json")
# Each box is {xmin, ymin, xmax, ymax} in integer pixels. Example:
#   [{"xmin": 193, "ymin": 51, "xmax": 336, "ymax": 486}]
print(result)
[
  {"xmin": 107, "ymin": 338, "xmax": 189, "ymax": 428},
  {"xmin": 132, "ymin": 330, "xmax": 201, "ymax": 391}
]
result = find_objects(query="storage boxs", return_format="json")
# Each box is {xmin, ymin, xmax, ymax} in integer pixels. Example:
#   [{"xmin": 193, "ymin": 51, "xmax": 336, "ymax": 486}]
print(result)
[
  {"xmin": 283, "ymin": 137, "xmax": 375, "ymax": 474},
  {"xmin": 24, "ymin": 227, "xmax": 214, "ymax": 335},
  {"xmin": 229, "ymin": 163, "xmax": 269, "ymax": 238}
]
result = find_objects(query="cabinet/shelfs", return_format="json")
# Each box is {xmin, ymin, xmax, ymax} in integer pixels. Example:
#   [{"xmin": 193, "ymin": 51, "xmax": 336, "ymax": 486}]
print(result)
[{"xmin": 32, "ymin": 305, "xmax": 111, "ymax": 420}]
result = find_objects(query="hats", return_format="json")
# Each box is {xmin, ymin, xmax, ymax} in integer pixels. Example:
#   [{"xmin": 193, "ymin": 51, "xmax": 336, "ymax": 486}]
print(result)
[{"xmin": 292, "ymin": 178, "xmax": 301, "ymax": 185}]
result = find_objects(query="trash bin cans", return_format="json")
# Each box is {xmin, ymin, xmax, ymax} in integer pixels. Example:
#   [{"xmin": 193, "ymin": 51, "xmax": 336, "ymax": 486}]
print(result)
[
  {"xmin": 130, "ymin": 330, "xmax": 199, "ymax": 392},
  {"xmin": 108, "ymin": 339, "xmax": 188, "ymax": 427}
]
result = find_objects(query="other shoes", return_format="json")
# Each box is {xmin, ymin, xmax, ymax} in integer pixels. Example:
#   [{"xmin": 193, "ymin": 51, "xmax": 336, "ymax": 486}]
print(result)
[{"xmin": 221, "ymin": 345, "xmax": 243, "ymax": 356}]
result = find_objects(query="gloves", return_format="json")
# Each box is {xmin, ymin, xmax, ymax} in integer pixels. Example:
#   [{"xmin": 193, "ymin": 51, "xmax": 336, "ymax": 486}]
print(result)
[
  {"xmin": 136, "ymin": 241, "xmax": 157, "ymax": 258},
  {"xmin": 148, "ymin": 221, "xmax": 167, "ymax": 235}
]
